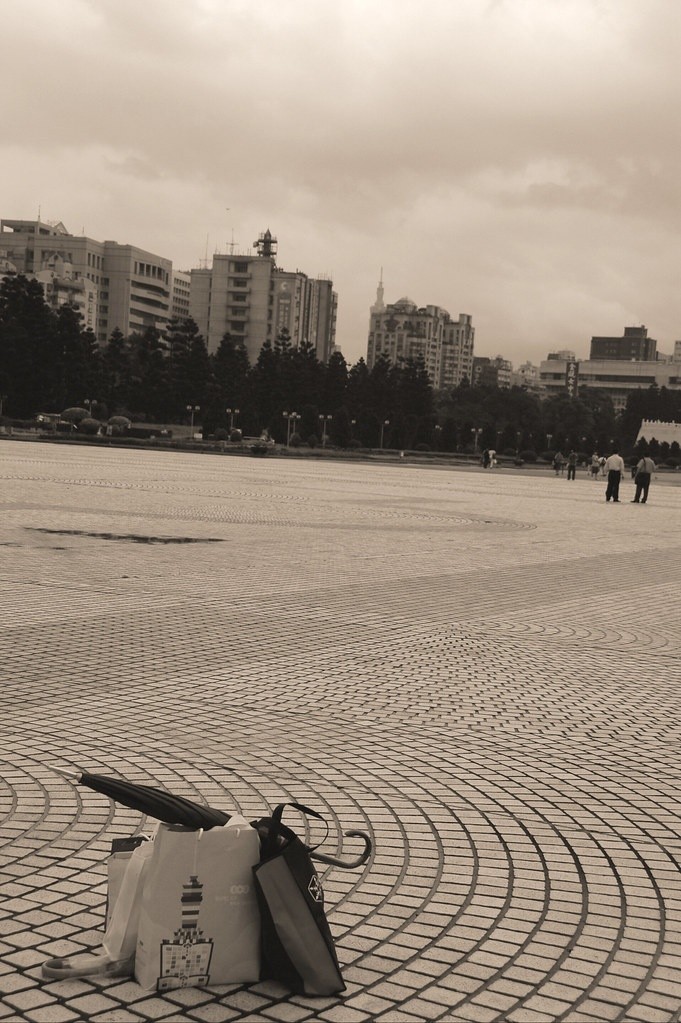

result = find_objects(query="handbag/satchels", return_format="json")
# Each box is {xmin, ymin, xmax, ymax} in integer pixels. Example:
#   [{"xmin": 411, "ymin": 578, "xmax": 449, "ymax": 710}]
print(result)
[
  {"xmin": 492, "ymin": 454, "xmax": 496, "ymax": 459},
  {"xmin": 101, "ymin": 803, "xmax": 348, "ymax": 998}
]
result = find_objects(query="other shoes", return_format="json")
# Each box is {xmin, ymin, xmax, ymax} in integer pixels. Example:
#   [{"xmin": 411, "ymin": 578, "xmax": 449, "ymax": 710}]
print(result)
[
  {"xmin": 630, "ymin": 501, "xmax": 639, "ymax": 503},
  {"xmin": 613, "ymin": 500, "xmax": 620, "ymax": 502},
  {"xmin": 606, "ymin": 499, "xmax": 610, "ymax": 501},
  {"xmin": 641, "ymin": 501, "xmax": 645, "ymax": 503}
]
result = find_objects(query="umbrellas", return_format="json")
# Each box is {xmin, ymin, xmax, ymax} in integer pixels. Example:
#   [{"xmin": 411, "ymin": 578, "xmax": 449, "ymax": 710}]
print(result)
[{"xmin": 46, "ymin": 764, "xmax": 372, "ymax": 869}]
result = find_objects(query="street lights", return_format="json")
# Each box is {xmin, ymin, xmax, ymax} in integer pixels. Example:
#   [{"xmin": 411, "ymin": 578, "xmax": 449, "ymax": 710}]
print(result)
[
  {"xmin": 471, "ymin": 428, "xmax": 483, "ymax": 453},
  {"xmin": 227, "ymin": 408, "xmax": 240, "ymax": 429},
  {"xmin": 381, "ymin": 420, "xmax": 389, "ymax": 449},
  {"xmin": 319, "ymin": 415, "xmax": 333, "ymax": 447},
  {"xmin": 187, "ymin": 405, "xmax": 200, "ymax": 437},
  {"xmin": 84, "ymin": 399, "xmax": 97, "ymax": 415},
  {"xmin": 283, "ymin": 411, "xmax": 297, "ymax": 448}
]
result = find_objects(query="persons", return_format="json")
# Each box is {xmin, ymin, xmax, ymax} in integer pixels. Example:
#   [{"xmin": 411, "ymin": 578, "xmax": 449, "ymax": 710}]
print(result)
[
  {"xmin": 552, "ymin": 448, "xmax": 576, "ymax": 481},
  {"xmin": 95, "ymin": 422, "xmax": 112, "ymax": 437},
  {"xmin": 629, "ymin": 451, "xmax": 656, "ymax": 504},
  {"xmin": 588, "ymin": 449, "xmax": 606, "ymax": 480},
  {"xmin": 603, "ymin": 448, "xmax": 624, "ymax": 502},
  {"xmin": 480, "ymin": 446, "xmax": 497, "ymax": 468}
]
